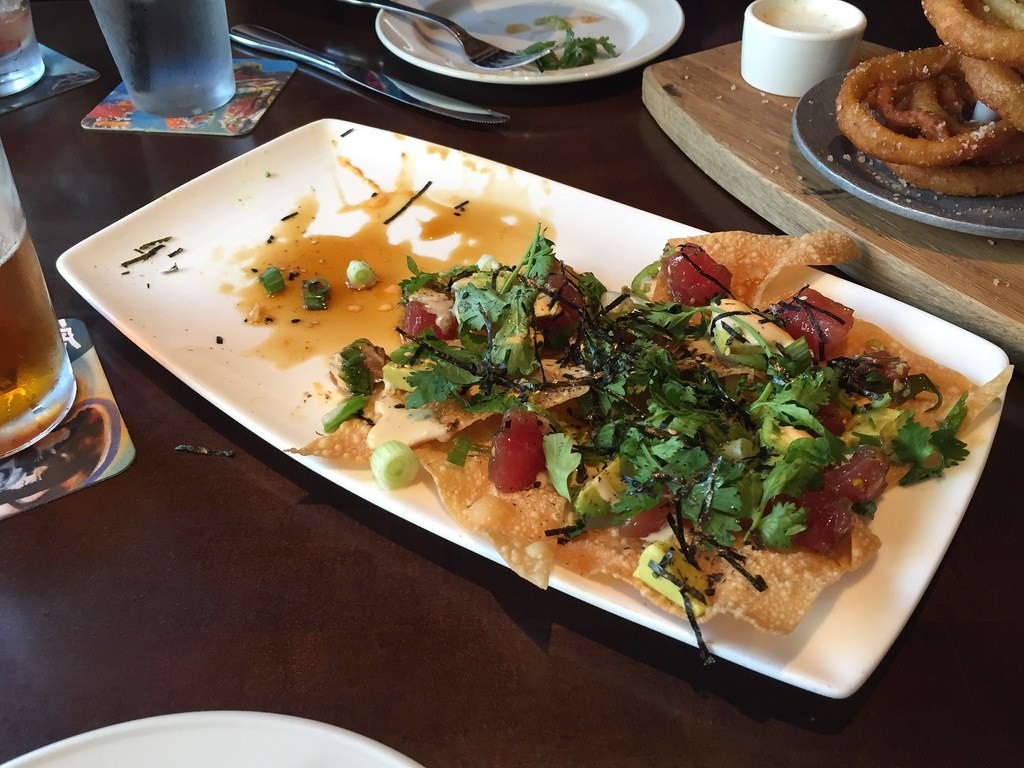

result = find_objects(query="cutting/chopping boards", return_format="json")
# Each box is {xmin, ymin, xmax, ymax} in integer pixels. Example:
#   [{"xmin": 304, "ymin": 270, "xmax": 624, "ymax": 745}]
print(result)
[{"xmin": 641, "ymin": 41, "xmax": 1023, "ymax": 374}]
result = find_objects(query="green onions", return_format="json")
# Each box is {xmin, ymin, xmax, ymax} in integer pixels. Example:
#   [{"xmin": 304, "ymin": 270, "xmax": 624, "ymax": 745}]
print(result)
[{"xmin": 259, "ymin": 260, "xmax": 375, "ymax": 310}]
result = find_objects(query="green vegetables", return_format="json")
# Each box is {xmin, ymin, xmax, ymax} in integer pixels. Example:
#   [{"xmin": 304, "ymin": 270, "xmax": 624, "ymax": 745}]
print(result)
[{"xmin": 323, "ymin": 222, "xmax": 969, "ymax": 549}]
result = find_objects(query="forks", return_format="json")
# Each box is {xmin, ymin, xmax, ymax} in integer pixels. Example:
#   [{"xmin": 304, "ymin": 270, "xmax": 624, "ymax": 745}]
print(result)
[{"xmin": 343, "ymin": 0, "xmax": 565, "ymax": 70}]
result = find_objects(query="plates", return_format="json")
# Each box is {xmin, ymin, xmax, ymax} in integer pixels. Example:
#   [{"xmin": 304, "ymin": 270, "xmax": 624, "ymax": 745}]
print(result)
[
  {"xmin": 792, "ymin": 73, "xmax": 1022, "ymax": 245},
  {"xmin": 4, "ymin": 709, "xmax": 423, "ymax": 768},
  {"xmin": 53, "ymin": 116, "xmax": 986, "ymax": 705},
  {"xmin": 373, "ymin": 2, "xmax": 685, "ymax": 88}
]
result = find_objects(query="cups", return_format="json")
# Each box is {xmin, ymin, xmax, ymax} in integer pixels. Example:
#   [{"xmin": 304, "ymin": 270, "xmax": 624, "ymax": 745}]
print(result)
[
  {"xmin": 88, "ymin": 3, "xmax": 237, "ymax": 115},
  {"xmin": 741, "ymin": 3, "xmax": 867, "ymax": 101},
  {"xmin": 1, "ymin": 2, "xmax": 46, "ymax": 97},
  {"xmin": 0, "ymin": 141, "xmax": 79, "ymax": 462}
]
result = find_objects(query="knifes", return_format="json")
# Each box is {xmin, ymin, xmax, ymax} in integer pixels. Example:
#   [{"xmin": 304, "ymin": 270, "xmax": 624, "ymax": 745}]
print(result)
[{"xmin": 227, "ymin": 22, "xmax": 509, "ymax": 126}]
